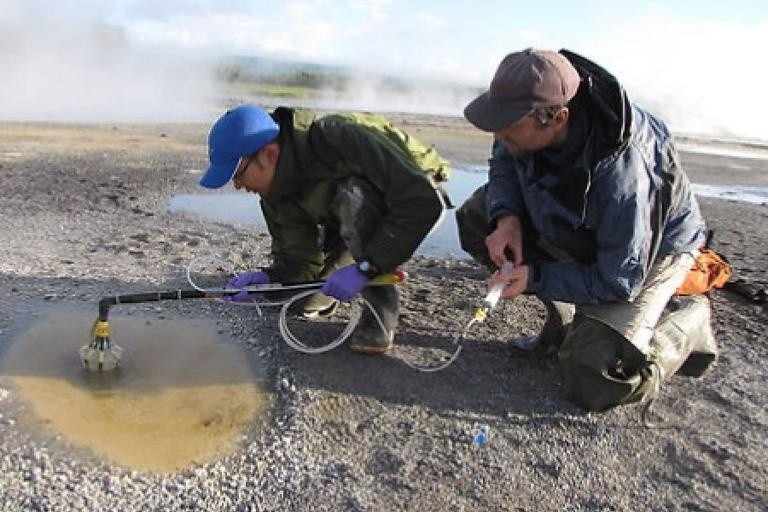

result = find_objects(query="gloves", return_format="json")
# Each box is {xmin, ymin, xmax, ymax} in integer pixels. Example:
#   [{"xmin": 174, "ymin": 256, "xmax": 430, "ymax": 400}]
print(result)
[
  {"xmin": 224, "ymin": 270, "xmax": 269, "ymax": 304},
  {"xmin": 322, "ymin": 260, "xmax": 369, "ymax": 303}
]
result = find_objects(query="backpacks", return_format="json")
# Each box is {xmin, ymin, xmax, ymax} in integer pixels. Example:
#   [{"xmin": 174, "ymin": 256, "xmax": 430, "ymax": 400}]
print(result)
[{"xmin": 671, "ymin": 241, "xmax": 732, "ymax": 296}]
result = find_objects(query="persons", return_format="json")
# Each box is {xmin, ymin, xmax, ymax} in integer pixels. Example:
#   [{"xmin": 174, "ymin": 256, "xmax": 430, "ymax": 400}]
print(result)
[
  {"xmin": 196, "ymin": 102, "xmax": 455, "ymax": 358},
  {"xmin": 453, "ymin": 44, "xmax": 732, "ymax": 413}
]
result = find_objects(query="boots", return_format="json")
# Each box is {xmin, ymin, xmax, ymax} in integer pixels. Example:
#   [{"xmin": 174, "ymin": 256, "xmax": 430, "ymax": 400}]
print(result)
[
  {"xmin": 642, "ymin": 289, "xmax": 719, "ymax": 382},
  {"xmin": 507, "ymin": 295, "xmax": 578, "ymax": 357},
  {"xmin": 349, "ymin": 274, "xmax": 400, "ymax": 352}
]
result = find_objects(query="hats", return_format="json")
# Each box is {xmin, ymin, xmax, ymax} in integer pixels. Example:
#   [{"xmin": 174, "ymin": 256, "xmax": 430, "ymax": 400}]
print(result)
[
  {"xmin": 200, "ymin": 104, "xmax": 280, "ymax": 189},
  {"xmin": 463, "ymin": 47, "xmax": 581, "ymax": 132}
]
